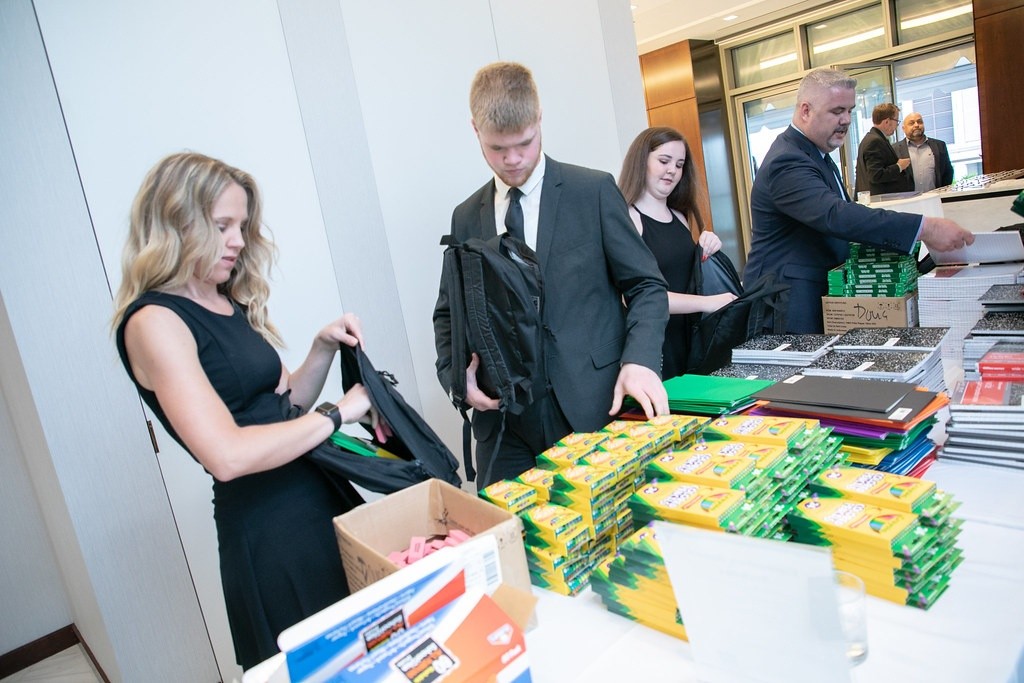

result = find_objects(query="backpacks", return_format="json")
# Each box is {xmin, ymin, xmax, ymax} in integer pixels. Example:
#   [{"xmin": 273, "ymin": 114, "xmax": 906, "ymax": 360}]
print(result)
[
  {"xmin": 682, "ymin": 241, "xmax": 793, "ymax": 375},
  {"xmin": 279, "ymin": 332, "xmax": 462, "ymax": 495},
  {"xmin": 439, "ymin": 232, "xmax": 545, "ymax": 415}
]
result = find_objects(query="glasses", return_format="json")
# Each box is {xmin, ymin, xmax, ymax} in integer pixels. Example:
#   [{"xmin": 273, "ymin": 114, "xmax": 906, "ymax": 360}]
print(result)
[{"xmin": 884, "ymin": 117, "xmax": 901, "ymax": 126}]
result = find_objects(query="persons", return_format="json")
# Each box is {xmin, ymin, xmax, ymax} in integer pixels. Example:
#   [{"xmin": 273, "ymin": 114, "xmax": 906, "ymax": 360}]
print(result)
[
  {"xmin": 890, "ymin": 113, "xmax": 953, "ymax": 193},
  {"xmin": 617, "ymin": 126, "xmax": 738, "ymax": 379},
  {"xmin": 433, "ymin": 62, "xmax": 669, "ymax": 496},
  {"xmin": 853, "ymin": 102, "xmax": 911, "ymax": 202},
  {"xmin": 742, "ymin": 67, "xmax": 974, "ymax": 336},
  {"xmin": 109, "ymin": 152, "xmax": 387, "ymax": 673}
]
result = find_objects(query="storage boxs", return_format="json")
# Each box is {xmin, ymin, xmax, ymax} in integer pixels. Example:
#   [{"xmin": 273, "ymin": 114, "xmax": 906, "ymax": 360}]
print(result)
[
  {"xmin": 276, "ymin": 417, "xmax": 965, "ymax": 683},
  {"xmin": 821, "ymin": 241, "xmax": 921, "ymax": 334}
]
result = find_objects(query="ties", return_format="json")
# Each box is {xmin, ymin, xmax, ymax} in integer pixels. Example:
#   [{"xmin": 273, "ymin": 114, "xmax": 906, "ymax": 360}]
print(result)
[
  {"xmin": 504, "ymin": 187, "xmax": 525, "ymax": 245},
  {"xmin": 824, "ymin": 153, "xmax": 843, "ymax": 200}
]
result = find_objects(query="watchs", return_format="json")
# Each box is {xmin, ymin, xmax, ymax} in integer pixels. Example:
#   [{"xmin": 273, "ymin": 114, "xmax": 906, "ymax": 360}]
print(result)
[{"xmin": 316, "ymin": 402, "xmax": 342, "ymax": 433}]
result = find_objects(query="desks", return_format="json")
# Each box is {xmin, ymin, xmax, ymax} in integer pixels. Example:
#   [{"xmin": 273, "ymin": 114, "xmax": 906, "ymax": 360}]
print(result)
[{"xmin": 243, "ymin": 355, "xmax": 1024, "ymax": 683}]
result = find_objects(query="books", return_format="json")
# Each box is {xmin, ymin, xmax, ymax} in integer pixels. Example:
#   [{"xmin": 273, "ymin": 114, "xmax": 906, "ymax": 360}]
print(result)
[{"xmin": 625, "ymin": 230, "xmax": 1024, "ymax": 479}]
